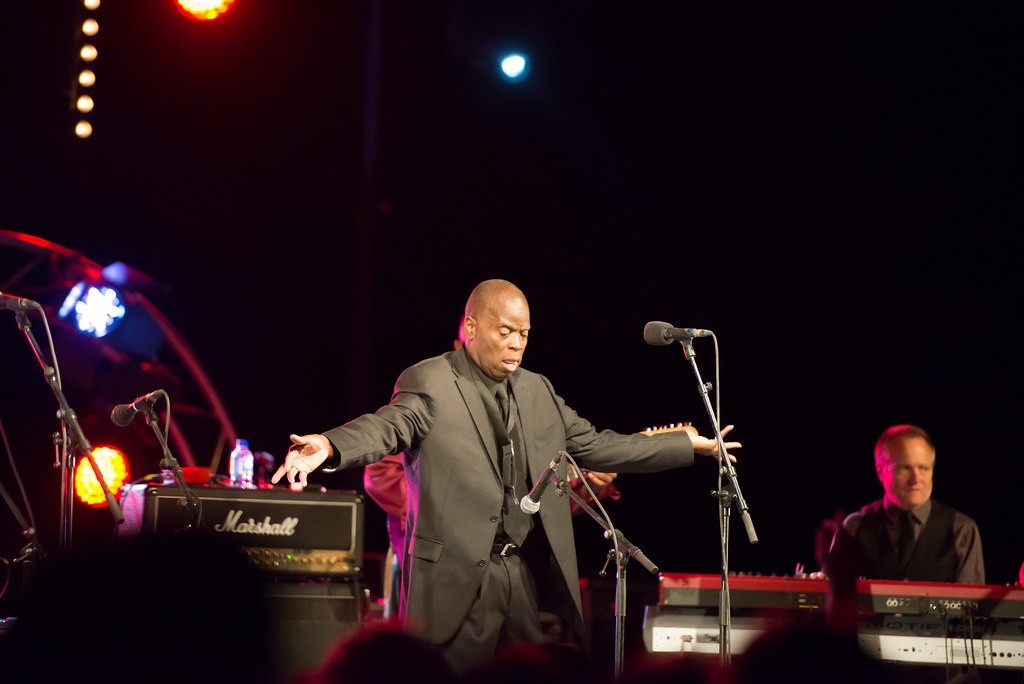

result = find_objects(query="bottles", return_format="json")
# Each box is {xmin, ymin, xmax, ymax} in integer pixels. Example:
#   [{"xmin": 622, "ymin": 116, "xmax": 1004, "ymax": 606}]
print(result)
[{"xmin": 229, "ymin": 439, "xmax": 254, "ymax": 488}]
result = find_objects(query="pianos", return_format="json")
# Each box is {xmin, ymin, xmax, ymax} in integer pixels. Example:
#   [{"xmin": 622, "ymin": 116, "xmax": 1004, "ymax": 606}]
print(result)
[{"xmin": 641, "ymin": 571, "xmax": 1023, "ymax": 671}]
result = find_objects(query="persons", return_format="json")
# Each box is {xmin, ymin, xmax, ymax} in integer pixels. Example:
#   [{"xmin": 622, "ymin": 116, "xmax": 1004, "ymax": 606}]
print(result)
[
  {"xmin": 825, "ymin": 424, "xmax": 985, "ymax": 622},
  {"xmin": 0, "ymin": 528, "xmax": 887, "ymax": 684},
  {"xmin": 269, "ymin": 280, "xmax": 742, "ymax": 684}
]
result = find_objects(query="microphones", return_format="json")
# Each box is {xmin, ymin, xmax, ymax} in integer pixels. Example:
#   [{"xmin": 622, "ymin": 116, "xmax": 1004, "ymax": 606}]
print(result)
[
  {"xmin": 521, "ymin": 452, "xmax": 563, "ymax": 515},
  {"xmin": 644, "ymin": 321, "xmax": 712, "ymax": 346},
  {"xmin": 0, "ymin": 293, "xmax": 41, "ymax": 310},
  {"xmin": 111, "ymin": 390, "xmax": 165, "ymax": 427}
]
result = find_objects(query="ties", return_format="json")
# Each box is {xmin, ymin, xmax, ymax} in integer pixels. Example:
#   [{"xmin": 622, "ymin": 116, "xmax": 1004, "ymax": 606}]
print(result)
[
  {"xmin": 493, "ymin": 384, "xmax": 532, "ymax": 548},
  {"xmin": 897, "ymin": 512, "xmax": 915, "ymax": 567}
]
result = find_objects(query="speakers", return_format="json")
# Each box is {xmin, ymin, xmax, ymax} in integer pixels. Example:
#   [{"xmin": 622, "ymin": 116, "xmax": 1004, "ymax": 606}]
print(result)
[{"xmin": 268, "ymin": 575, "xmax": 363, "ymax": 674}]
